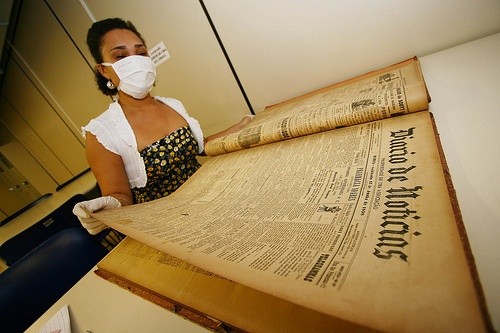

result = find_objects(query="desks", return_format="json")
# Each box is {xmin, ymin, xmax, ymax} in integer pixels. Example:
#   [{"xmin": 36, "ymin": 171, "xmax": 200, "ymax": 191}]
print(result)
[{"xmin": 25, "ymin": 32, "xmax": 500, "ymax": 333}]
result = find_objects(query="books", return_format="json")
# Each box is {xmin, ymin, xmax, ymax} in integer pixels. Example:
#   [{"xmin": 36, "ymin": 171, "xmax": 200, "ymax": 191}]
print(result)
[{"xmin": 81, "ymin": 56, "xmax": 494, "ymax": 333}]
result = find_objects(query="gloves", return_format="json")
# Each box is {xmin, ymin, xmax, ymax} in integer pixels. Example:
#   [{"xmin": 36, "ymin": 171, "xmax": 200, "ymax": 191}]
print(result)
[{"xmin": 73, "ymin": 196, "xmax": 122, "ymax": 236}]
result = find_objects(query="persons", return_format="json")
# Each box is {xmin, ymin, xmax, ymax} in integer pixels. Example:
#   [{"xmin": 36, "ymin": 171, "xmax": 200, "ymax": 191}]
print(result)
[{"xmin": 72, "ymin": 18, "xmax": 255, "ymax": 236}]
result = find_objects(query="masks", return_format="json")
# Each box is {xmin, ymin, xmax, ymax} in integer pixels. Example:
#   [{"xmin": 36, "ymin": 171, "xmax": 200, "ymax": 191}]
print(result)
[{"xmin": 103, "ymin": 54, "xmax": 157, "ymax": 100}]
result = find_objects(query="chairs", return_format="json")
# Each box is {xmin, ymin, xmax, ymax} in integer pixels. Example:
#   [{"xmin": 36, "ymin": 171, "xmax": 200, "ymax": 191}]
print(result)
[{"xmin": 0, "ymin": 185, "xmax": 125, "ymax": 333}]
